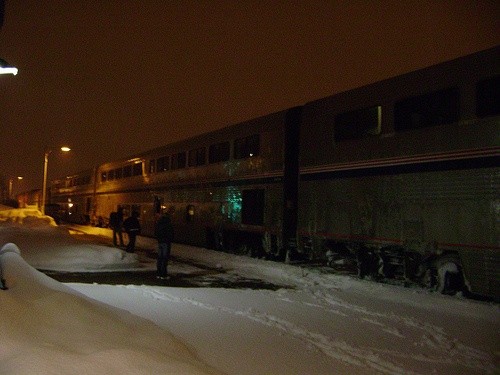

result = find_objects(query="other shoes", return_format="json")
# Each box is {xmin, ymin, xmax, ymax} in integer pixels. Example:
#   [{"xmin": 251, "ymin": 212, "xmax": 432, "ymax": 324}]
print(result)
[{"xmin": 157, "ymin": 275, "xmax": 170, "ymax": 279}]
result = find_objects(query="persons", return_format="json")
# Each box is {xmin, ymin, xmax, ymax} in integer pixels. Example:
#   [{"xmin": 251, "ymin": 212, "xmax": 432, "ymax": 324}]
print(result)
[
  {"xmin": 110, "ymin": 207, "xmax": 141, "ymax": 254},
  {"xmin": 404, "ymin": 239, "xmax": 440, "ymax": 295},
  {"xmin": 154, "ymin": 205, "xmax": 176, "ymax": 280}
]
